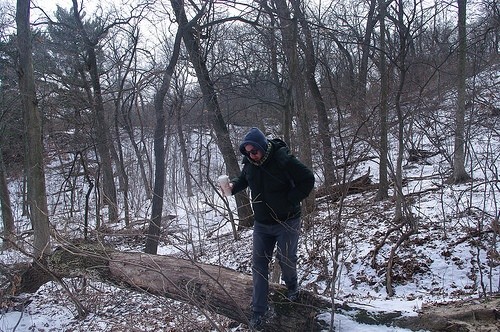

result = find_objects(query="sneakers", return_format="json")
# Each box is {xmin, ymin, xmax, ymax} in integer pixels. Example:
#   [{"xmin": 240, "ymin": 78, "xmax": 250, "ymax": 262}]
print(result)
[
  {"xmin": 288, "ymin": 286, "xmax": 300, "ymax": 301},
  {"xmin": 248, "ymin": 312, "xmax": 265, "ymax": 332}
]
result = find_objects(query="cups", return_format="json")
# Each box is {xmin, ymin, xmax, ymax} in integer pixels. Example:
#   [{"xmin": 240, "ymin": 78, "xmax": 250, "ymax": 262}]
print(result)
[{"xmin": 218, "ymin": 174, "xmax": 232, "ymax": 196}]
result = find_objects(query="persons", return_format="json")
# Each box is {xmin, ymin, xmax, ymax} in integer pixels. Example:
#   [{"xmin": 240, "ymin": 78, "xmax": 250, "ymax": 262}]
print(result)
[{"xmin": 220, "ymin": 127, "xmax": 318, "ymax": 327}]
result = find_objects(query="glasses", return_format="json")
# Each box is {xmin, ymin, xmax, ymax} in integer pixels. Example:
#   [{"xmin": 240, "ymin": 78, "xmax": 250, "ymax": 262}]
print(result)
[{"xmin": 244, "ymin": 149, "xmax": 258, "ymax": 156}]
